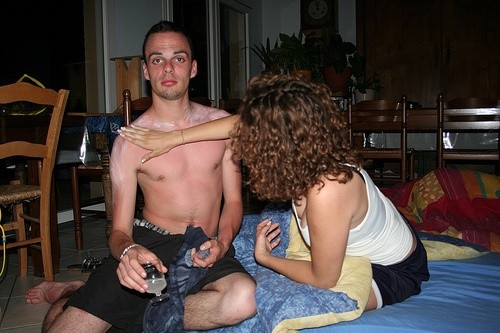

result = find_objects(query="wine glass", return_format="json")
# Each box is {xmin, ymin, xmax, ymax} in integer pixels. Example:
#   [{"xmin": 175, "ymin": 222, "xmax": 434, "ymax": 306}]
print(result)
[{"xmin": 140, "ymin": 259, "xmax": 171, "ymax": 304}]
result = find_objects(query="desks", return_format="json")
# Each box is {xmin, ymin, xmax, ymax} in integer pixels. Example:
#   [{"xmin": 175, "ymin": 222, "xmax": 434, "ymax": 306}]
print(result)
[
  {"xmin": 344, "ymin": 109, "xmax": 500, "ymax": 181},
  {"xmin": 1, "ymin": 110, "xmax": 124, "ymax": 276}
]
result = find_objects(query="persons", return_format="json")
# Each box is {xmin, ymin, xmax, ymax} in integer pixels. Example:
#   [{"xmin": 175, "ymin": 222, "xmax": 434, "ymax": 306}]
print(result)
[
  {"xmin": 116, "ymin": 72, "xmax": 429, "ymax": 314},
  {"xmin": 26, "ymin": 20, "xmax": 258, "ymax": 333}
]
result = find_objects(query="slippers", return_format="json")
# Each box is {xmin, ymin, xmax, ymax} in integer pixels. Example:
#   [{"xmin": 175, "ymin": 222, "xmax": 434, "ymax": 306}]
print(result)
[{"xmin": 66, "ymin": 256, "xmax": 108, "ymax": 275}]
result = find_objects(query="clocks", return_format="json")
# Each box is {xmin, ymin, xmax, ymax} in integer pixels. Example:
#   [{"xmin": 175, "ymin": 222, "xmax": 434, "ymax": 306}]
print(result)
[{"xmin": 300, "ymin": 0, "xmax": 340, "ymax": 37}]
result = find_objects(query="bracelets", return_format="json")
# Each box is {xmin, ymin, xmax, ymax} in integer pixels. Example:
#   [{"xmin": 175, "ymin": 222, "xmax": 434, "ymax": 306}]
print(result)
[
  {"xmin": 120, "ymin": 244, "xmax": 142, "ymax": 259},
  {"xmin": 181, "ymin": 130, "xmax": 184, "ymax": 143}
]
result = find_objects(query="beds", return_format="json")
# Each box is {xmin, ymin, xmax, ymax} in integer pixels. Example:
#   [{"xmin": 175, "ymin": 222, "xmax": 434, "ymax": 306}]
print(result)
[{"xmin": 200, "ymin": 204, "xmax": 500, "ymax": 333}]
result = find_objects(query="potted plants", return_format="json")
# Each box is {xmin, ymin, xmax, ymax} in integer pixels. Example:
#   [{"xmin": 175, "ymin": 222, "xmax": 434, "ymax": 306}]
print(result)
[{"xmin": 245, "ymin": 32, "xmax": 384, "ymax": 104}]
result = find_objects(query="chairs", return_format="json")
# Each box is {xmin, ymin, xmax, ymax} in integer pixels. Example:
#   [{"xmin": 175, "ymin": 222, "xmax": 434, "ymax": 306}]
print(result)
[
  {"xmin": 72, "ymin": 89, "xmax": 152, "ymax": 252},
  {"xmin": 345, "ymin": 85, "xmax": 416, "ymax": 183},
  {"xmin": 436, "ymin": 93, "xmax": 500, "ymax": 171},
  {"xmin": 0, "ymin": 82, "xmax": 72, "ymax": 282},
  {"xmin": 219, "ymin": 98, "xmax": 251, "ymax": 204}
]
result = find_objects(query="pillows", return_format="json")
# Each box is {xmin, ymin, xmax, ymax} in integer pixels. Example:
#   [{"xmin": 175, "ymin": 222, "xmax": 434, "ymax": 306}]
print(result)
[{"xmin": 387, "ymin": 167, "xmax": 500, "ymax": 253}]
